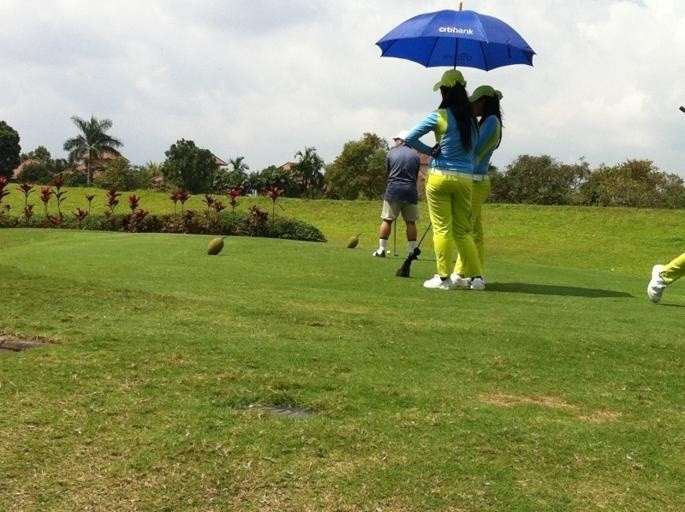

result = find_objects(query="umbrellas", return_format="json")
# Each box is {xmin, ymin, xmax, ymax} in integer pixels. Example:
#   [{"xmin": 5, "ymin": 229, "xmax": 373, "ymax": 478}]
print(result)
[{"xmin": 375, "ymin": 2, "xmax": 537, "ymax": 71}]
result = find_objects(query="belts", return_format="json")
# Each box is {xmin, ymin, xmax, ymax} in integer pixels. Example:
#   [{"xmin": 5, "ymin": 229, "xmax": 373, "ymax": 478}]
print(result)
[
  {"xmin": 427, "ymin": 167, "xmax": 473, "ymax": 179},
  {"xmin": 472, "ymin": 175, "xmax": 490, "ymax": 181}
]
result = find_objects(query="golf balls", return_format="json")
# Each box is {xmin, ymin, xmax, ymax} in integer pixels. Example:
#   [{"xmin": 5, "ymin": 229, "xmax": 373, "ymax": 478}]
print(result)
[{"xmin": 387, "ymin": 251, "xmax": 390, "ymax": 254}]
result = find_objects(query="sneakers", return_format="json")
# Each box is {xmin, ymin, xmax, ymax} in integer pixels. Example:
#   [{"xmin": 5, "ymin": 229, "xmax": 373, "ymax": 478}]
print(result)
[
  {"xmin": 449, "ymin": 272, "xmax": 471, "ymax": 288},
  {"xmin": 469, "ymin": 277, "xmax": 486, "ymax": 290},
  {"xmin": 422, "ymin": 274, "xmax": 454, "ymax": 290},
  {"xmin": 372, "ymin": 249, "xmax": 387, "ymax": 257},
  {"xmin": 645, "ymin": 263, "xmax": 668, "ymax": 304},
  {"xmin": 408, "ymin": 251, "xmax": 418, "ymax": 260}
]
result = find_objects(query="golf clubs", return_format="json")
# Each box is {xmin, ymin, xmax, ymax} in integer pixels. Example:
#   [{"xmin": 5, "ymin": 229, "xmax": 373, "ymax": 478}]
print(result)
[{"xmin": 394, "ymin": 219, "xmax": 399, "ymax": 256}]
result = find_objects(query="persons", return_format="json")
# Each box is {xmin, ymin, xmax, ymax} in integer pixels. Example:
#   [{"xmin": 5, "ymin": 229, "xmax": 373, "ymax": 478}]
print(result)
[
  {"xmin": 646, "ymin": 253, "xmax": 685, "ymax": 303},
  {"xmin": 373, "ymin": 133, "xmax": 421, "ymax": 258},
  {"xmin": 450, "ymin": 86, "xmax": 506, "ymax": 286},
  {"xmin": 406, "ymin": 69, "xmax": 486, "ymax": 291}
]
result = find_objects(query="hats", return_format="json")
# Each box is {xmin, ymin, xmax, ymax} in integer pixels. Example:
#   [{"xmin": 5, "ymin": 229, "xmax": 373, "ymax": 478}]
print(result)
[
  {"xmin": 467, "ymin": 85, "xmax": 503, "ymax": 103},
  {"xmin": 433, "ymin": 69, "xmax": 467, "ymax": 92},
  {"xmin": 392, "ymin": 131, "xmax": 408, "ymax": 143}
]
